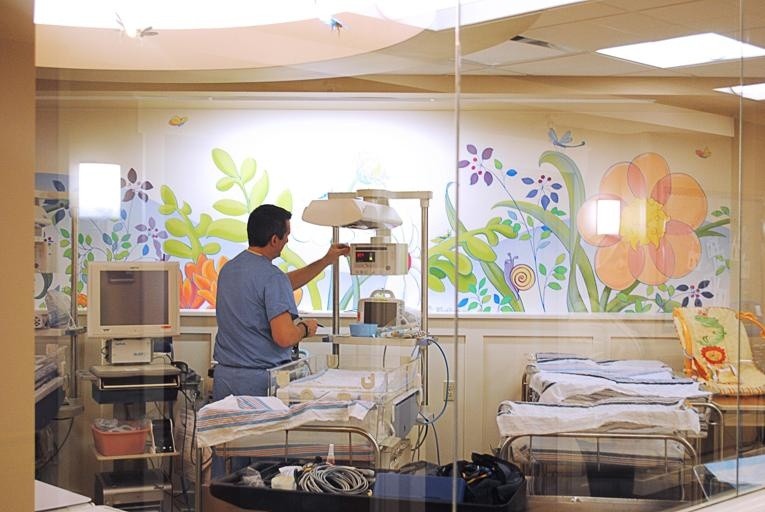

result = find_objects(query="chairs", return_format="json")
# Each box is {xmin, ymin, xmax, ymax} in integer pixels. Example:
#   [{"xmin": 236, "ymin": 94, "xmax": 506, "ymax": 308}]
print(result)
[{"xmin": 672, "ymin": 306, "xmax": 765, "ymax": 460}]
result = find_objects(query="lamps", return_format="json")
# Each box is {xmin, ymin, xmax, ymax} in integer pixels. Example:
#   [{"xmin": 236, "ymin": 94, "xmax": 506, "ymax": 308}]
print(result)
[
  {"xmin": 594, "ymin": 195, "xmax": 623, "ymax": 237},
  {"xmin": 76, "ymin": 163, "xmax": 122, "ymax": 219}
]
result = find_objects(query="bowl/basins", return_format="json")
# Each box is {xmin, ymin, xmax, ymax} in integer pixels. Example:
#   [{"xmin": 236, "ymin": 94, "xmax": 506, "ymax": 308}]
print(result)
[{"xmin": 349, "ymin": 323, "xmax": 378, "ymax": 337}]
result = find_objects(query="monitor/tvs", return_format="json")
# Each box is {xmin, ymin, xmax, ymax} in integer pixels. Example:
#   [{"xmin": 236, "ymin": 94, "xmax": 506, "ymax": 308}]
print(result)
[{"xmin": 87, "ymin": 262, "xmax": 180, "ymax": 338}]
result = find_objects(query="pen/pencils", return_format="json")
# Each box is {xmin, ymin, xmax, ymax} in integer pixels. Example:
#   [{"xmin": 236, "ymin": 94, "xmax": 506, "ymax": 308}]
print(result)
[{"xmin": 299, "ymin": 317, "xmax": 324, "ymax": 328}]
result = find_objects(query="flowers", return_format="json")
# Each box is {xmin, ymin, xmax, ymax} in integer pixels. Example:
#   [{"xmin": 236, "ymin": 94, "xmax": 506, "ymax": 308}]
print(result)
[{"xmin": 492, "ymin": 151, "xmax": 732, "ymax": 314}]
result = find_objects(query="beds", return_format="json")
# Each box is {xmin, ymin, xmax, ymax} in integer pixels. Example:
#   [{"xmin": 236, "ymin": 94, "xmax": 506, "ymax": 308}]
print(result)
[{"xmin": 520, "ymin": 369, "xmax": 724, "ymax": 495}]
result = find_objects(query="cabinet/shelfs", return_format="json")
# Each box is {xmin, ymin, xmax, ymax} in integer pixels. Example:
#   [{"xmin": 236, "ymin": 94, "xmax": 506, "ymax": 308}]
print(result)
[{"xmin": 32, "ymin": 170, "xmax": 85, "ymax": 491}]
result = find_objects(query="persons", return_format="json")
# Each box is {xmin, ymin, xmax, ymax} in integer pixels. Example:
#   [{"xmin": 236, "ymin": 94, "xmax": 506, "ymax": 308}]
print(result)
[{"xmin": 210, "ymin": 205, "xmax": 350, "ymax": 480}]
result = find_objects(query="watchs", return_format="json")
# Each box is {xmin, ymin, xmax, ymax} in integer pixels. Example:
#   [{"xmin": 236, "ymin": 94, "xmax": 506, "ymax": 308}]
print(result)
[{"xmin": 298, "ymin": 321, "xmax": 312, "ymax": 339}]
